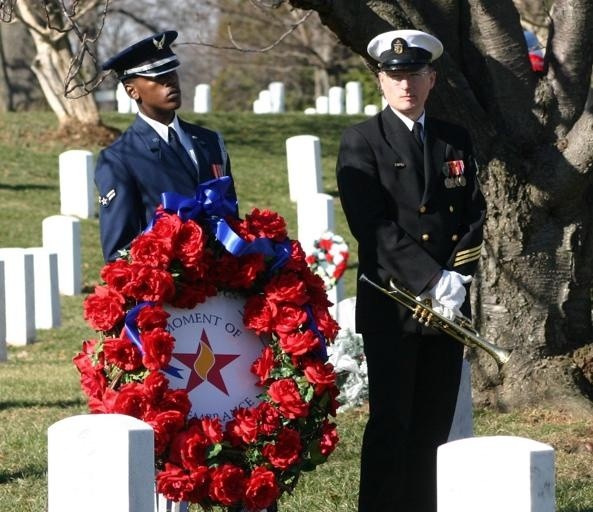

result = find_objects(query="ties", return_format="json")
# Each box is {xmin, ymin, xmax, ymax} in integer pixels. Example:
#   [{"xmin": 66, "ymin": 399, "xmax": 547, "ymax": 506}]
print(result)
[
  {"xmin": 167, "ymin": 128, "xmax": 198, "ymax": 179},
  {"xmin": 412, "ymin": 122, "xmax": 424, "ymax": 150}
]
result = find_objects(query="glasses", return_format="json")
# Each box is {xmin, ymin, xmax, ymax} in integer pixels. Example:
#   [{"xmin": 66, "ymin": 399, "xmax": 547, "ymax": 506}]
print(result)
[{"xmin": 387, "ymin": 71, "xmax": 429, "ymax": 85}]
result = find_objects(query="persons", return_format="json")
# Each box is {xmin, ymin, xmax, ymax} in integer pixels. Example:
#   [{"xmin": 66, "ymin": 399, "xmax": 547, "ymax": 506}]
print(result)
[
  {"xmin": 94, "ymin": 31, "xmax": 238, "ymax": 266},
  {"xmin": 335, "ymin": 29, "xmax": 487, "ymax": 512}
]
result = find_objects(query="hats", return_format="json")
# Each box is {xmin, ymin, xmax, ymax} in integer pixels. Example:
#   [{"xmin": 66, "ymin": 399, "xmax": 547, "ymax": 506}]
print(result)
[
  {"xmin": 366, "ymin": 29, "xmax": 443, "ymax": 75},
  {"xmin": 101, "ymin": 30, "xmax": 182, "ymax": 81}
]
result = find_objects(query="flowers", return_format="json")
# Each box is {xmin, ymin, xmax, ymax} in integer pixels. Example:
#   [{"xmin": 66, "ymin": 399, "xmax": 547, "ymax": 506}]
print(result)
[{"xmin": 72, "ymin": 205, "xmax": 350, "ymax": 511}]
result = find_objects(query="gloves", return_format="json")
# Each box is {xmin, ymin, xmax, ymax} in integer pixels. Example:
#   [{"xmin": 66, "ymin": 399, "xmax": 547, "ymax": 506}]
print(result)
[
  {"xmin": 416, "ymin": 291, "xmax": 455, "ymax": 323},
  {"xmin": 429, "ymin": 270, "xmax": 473, "ymax": 316}
]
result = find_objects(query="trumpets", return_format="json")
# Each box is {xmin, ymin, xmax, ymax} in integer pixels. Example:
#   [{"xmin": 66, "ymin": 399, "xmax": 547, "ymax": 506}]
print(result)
[{"xmin": 358, "ymin": 273, "xmax": 515, "ymax": 373}]
